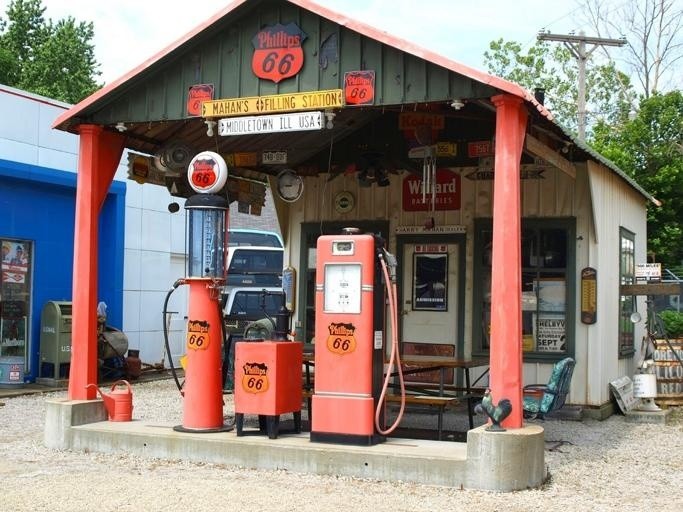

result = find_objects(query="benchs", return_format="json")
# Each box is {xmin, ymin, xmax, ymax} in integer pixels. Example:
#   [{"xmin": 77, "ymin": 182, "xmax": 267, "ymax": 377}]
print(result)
[
  {"xmin": 298, "ymin": 390, "xmax": 459, "ymax": 442},
  {"xmin": 383, "ymin": 380, "xmax": 487, "ymax": 430}
]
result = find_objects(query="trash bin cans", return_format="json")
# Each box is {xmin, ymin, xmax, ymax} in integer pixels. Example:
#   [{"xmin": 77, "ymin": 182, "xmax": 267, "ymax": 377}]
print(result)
[{"xmin": 39, "ymin": 301, "xmax": 72, "ymax": 380}]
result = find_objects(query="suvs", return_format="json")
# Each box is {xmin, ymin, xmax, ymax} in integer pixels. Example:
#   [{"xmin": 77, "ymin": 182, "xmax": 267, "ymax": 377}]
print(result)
[
  {"xmin": 207, "ymin": 228, "xmax": 283, "ymax": 263},
  {"xmin": 220, "ymin": 286, "xmax": 287, "ymax": 356},
  {"xmin": 207, "ymin": 246, "xmax": 285, "ymax": 287}
]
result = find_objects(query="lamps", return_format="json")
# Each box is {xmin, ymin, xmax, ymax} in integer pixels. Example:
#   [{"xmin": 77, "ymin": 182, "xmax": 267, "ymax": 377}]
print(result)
[{"xmin": 204, "ymin": 107, "xmax": 338, "ymax": 138}]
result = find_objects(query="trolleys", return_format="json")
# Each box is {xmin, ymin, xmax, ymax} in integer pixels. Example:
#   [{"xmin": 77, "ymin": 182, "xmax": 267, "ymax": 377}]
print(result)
[{"xmin": 139, "ymin": 309, "xmax": 178, "ymax": 375}]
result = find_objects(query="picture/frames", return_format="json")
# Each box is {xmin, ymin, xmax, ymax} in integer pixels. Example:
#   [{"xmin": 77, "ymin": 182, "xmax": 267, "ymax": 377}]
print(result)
[{"xmin": 410, "ymin": 251, "xmax": 448, "ymax": 312}]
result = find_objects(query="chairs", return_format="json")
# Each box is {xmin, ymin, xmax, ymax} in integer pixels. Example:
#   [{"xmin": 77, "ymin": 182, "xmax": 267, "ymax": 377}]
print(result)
[{"xmin": 522, "ymin": 355, "xmax": 577, "ymax": 451}]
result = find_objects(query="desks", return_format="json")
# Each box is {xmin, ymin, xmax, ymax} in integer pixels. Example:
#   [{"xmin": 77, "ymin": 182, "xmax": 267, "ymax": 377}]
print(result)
[{"xmin": 301, "ymin": 352, "xmax": 487, "ymax": 432}]
